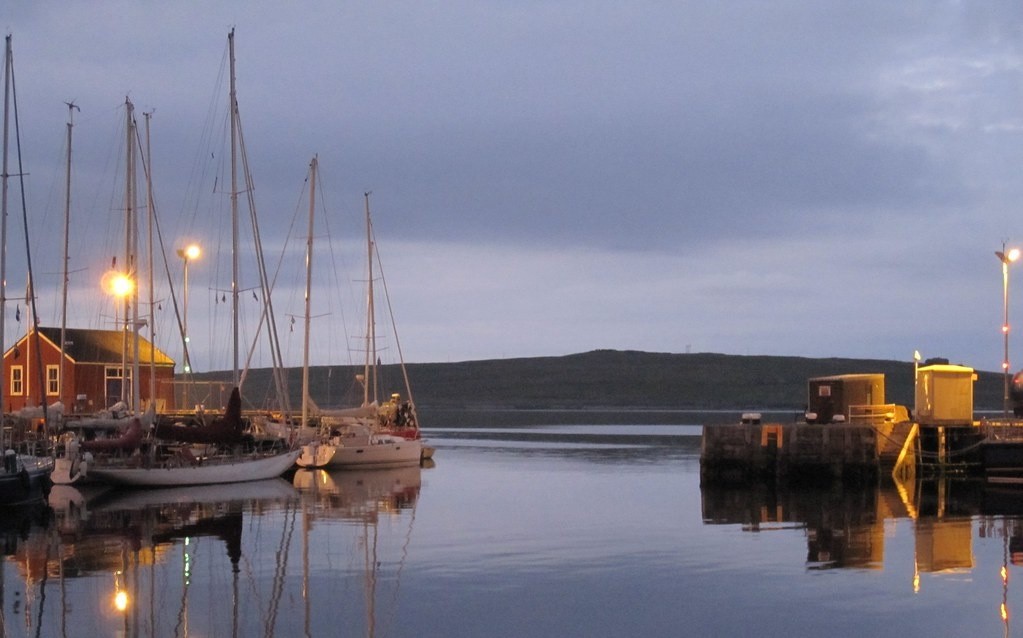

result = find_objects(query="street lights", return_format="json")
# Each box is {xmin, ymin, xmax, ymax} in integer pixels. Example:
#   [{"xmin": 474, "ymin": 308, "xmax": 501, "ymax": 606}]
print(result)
[
  {"xmin": 112, "ymin": 574, "xmax": 127, "ymax": 611},
  {"xmin": 178, "ymin": 243, "xmax": 201, "ymax": 412},
  {"xmin": 112, "ymin": 275, "xmax": 132, "ymax": 409},
  {"xmin": 1004, "ymin": 248, "xmax": 1020, "ymax": 437}
]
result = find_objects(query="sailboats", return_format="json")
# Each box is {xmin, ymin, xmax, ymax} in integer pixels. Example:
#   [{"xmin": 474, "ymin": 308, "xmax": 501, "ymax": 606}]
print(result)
[
  {"xmin": 309, "ymin": 465, "xmax": 423, "ymax": 638},
  {"xmin": 84, "ymin": 475, "xmax": 297, "ymax": 638},
  {"xmin": 0, "ymin": 22, "xmax": 439, "ymax": 493}
]
